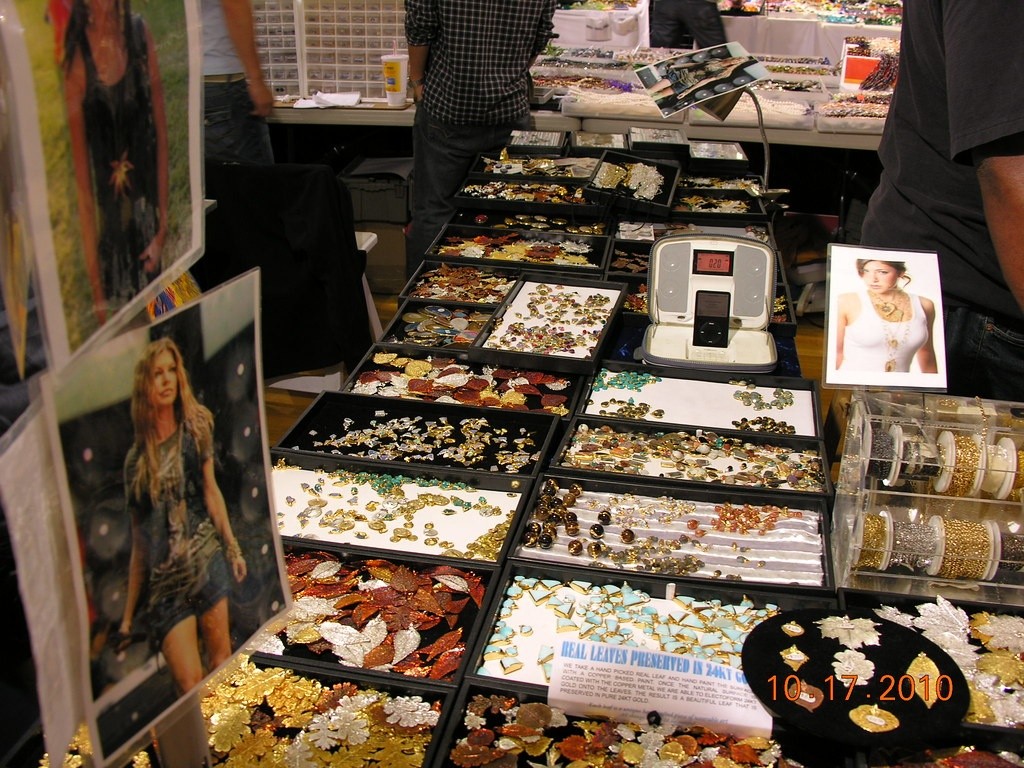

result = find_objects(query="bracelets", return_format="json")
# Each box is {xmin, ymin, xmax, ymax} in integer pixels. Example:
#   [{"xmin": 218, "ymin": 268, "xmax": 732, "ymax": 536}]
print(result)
[
  {"xmin": 224, "ymin": 539, "xmax": 241, "ymax": 559},
  {"xmin": 408, "ymin": 76, "xmax": 426, "ymax": 88},
  {"xmin": 245, "ymin": 76, "xmax": 266, "ymax": 84}
]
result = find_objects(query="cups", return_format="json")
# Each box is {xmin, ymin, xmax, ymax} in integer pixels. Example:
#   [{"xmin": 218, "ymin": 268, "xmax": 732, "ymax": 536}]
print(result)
[
  {"xmin": 586, "ymin": 15, "xmax": 613, "ymax": 40},
  {"xmin": 382, "ymin": 54, "xmax": 409, "ymax": 106}
]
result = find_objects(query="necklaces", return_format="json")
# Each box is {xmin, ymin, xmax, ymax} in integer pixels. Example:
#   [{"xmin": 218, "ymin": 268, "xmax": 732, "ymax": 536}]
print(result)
[{"xmin": 871, "ymin": 291, "xmax": 911, "ymax": 372}]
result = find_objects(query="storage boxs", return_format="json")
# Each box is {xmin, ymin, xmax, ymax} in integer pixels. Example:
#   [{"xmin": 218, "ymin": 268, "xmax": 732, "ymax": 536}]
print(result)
[{"xmin": 103, "ymin": 53, "xmax": 1024, "ymax": 767}]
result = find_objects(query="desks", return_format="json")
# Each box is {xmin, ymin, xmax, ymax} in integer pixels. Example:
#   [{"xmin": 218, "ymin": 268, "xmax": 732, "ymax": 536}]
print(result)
[
  {"xmin": 583, "ymin": 117, "xmax": 890, "ymax": 244},
  {"xmin": 263, "ymin": 94, "xmax": 420, "ymax": 123},
  {"xmin": 552, "ymin": 11, "xmax": 907, "ymax": 58}
]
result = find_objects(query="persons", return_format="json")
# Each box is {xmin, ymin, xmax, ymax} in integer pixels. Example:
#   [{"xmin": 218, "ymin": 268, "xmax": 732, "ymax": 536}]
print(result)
[
  {"xmin": 198, "ymin": 0, "xmax": 274, "ymax": 164},
  {"xmin": 61, "ymin": 0, "xmax": 168, "ymax": 322},
  {"xmin": 403, "ymin": 0, "xmax": 558, "ymax": 273},
  {"xmin": 118, "ymin": 338, "xmax": 248, "ymax": 696},
  {"xmin": 652, "ymin": 0, "xmax": 727, "ymax": 51},
  {"xmin": 861, "ymin": 0, "xmax": 1024, "ymax": 401},
  {"xmin": 647, "ymin": 56, "xmax": 748, "ymax": 100},
  {"xmin": 836, "ymin": 259, "xmax": 937, "ymax": 373}
]
